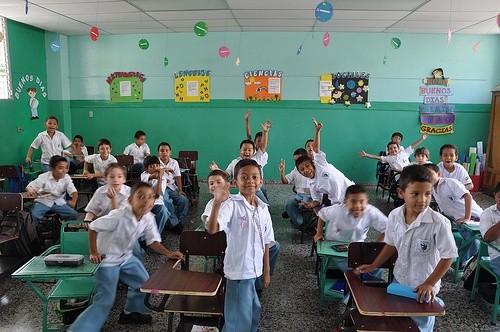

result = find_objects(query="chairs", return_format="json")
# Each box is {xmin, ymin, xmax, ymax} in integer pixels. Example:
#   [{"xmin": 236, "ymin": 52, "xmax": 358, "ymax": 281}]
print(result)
[
  {"xmin": 0, "ymin": 144, "xmax": 226, "ymax": 332},
  {"xmin": 293, "ymin": 151, "xmax": 500, "ymax": 332}
]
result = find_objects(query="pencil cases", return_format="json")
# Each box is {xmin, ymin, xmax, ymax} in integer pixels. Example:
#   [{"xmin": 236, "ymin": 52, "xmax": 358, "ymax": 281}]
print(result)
[
  {"xmin": 387, "ymin": 283, "xmax": 426, "ymax": 301},
  {"xmin": 332, "ymin": 244, "xmax": 349, "ymax": 251},
  {"xmin": 44, "ymin": 254, "xmax": 84, "ymax": 266}
]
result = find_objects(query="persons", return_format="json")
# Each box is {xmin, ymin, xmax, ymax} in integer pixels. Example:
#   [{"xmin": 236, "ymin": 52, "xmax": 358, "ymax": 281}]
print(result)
[{"xmin": 25, "ymin": 110, "xmax": 500, "ymax": 332}]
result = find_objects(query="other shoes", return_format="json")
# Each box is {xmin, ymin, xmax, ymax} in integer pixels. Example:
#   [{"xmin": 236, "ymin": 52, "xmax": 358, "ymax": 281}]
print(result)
[{"xmin": 119, "ymin": 311, "xmax": 153, "ymax": 324}]
